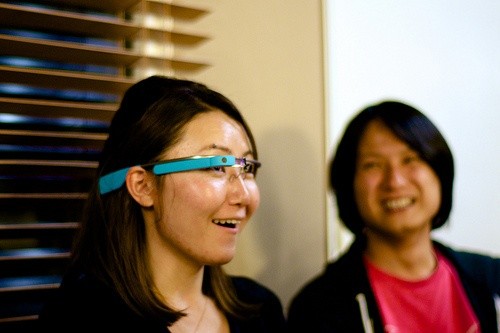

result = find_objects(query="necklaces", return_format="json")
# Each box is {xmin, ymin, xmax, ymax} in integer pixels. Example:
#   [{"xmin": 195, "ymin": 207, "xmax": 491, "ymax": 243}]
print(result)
[{"xmin": 152, "ymin": 287, "xmax": 213, "ymax": 333}]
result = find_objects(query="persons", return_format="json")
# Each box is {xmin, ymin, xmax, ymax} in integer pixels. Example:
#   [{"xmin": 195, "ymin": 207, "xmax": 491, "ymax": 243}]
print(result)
[
  {"xmin": 286, "ymin": 101, "xmax": 498, "ymax": 333},
  {"xmin": 35, "ymin": 75, "xmax": 287, "ymax": 333}
]
